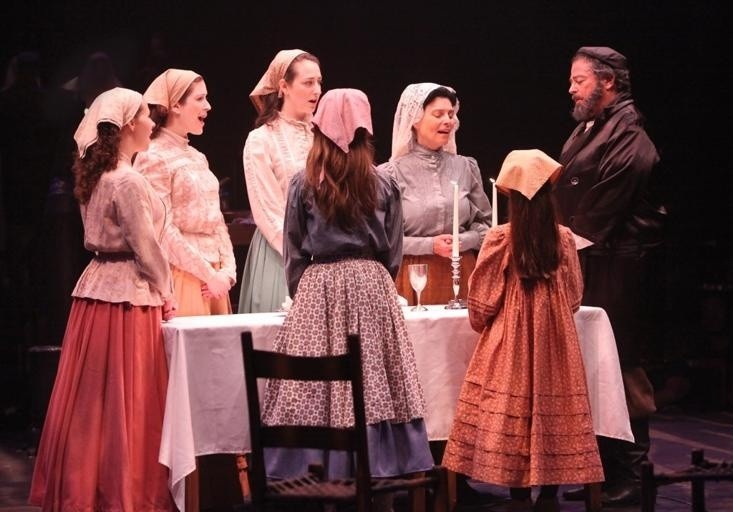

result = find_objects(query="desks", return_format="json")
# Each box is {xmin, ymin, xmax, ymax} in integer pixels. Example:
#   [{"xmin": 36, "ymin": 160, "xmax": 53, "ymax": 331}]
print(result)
[{"xmin": 161, "ymin": 300, "xmax": 604, "ymax": 512}]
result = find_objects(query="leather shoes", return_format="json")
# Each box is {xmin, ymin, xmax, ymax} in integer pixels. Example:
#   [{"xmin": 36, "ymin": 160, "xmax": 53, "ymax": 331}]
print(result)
[{"xmin": 564, "ymin": 480, "xmax": 642, "ymax": 506}]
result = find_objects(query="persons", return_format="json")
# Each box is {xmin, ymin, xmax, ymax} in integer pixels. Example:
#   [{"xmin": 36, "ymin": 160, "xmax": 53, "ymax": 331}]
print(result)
[
  {"xmin": 132, "ymin": 68, "xmax": 251, "ymax": 507},
  {"xmin": 439, "ymin": 146, "xmax": 606, "ymax": 512},
  {"xmin": 235, "ymin": 48, "xmax": 325, "ymax": 311},
  {"xmin": 25, "ymin": 86, "xmax": 180, "ymax": 512},
  {"xmin": 373, "ymin": 81, "xmax": 492, "ymax": 307},
  {"xmin": 258, "ymin": 87, "xmax": 434, "ymax": 485},
  {"xmin": 548, "ymin": 45, "xmax": 662, "ymax": 506}
]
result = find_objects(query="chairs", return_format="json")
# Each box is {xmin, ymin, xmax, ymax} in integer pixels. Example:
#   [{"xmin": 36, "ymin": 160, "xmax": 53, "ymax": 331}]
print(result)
[{"xmin": 240, "ymin": 330, "xmax": 437, "ymax": 512}]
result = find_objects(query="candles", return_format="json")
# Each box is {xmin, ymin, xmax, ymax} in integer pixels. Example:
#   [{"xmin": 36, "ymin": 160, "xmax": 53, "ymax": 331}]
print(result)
[{"xmin": 452, "ymin": 182, "xmax": 498, "ymax": 256}]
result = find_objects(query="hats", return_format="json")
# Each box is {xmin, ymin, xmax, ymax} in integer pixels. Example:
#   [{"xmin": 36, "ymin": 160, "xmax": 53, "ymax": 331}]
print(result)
[{"xmin": 578, "ymin": 47, "xmax": 626, "ymax": 68}]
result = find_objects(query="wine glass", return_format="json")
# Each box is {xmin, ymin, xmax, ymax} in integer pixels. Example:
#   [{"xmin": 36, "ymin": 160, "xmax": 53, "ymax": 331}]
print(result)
[{"xmin": 407, "ymin": 263, "xmax": 428, "ymax": 312}]
